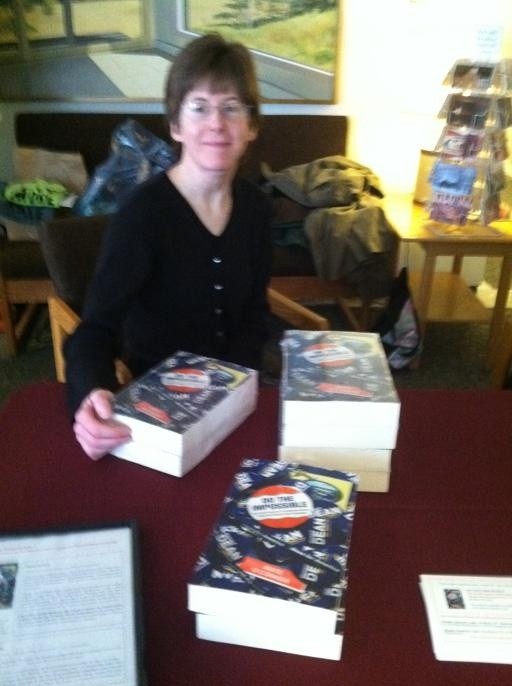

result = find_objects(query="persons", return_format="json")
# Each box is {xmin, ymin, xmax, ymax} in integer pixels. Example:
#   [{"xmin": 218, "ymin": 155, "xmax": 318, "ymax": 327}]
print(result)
[{"xmin": 62, "ymin": 32, "xmax": 282, "ymax": 462}]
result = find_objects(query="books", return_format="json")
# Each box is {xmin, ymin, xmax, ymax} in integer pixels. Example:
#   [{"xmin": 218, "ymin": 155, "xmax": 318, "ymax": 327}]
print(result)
[
  {"xmin": 193, "ymin": 590, "xmax": 349, "ymax": 663},
  {"xmin": 185, "ymin": 452, "xmax": 356, "ymax": 633},
  {"xmin": 272, "ymin": 323, "xmax": 402, "ymax": 497},
  {"xmin": 95, "ymin": 345, "xmax": 263, "ymax": 478}
]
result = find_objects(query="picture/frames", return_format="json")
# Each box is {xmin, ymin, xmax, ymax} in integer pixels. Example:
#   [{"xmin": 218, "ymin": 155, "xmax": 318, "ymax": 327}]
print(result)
[{"xmin": 2, "ymin": 0, "xmax": 345, "ymax": 107}]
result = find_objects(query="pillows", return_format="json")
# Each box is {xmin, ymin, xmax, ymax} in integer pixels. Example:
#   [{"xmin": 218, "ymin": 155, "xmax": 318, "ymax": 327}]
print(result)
[{"xmin": 266, "ymin": 155, "xmax": 383, "ymax": 209}]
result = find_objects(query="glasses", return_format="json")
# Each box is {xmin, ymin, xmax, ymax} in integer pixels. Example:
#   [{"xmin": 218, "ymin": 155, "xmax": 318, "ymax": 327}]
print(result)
[{"xmin": 176, "ymin": 99, "xmax": 258, "ymax": 121}]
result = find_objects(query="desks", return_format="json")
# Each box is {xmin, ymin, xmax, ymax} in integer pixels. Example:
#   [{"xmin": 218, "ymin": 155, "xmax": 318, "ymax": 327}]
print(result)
[
  {"xmin": 0, "ymin": 382, "xmax": 510, "ymax": 684},
  {"xmin": 369, "ymin": 185, "xmax": 511, "ymax": 375}
]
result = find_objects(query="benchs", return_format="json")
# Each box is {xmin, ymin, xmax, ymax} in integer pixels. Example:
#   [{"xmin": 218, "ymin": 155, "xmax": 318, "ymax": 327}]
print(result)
[{"xmin": 1, "ymin": 108, "xmax": 374, "ymax": 358}]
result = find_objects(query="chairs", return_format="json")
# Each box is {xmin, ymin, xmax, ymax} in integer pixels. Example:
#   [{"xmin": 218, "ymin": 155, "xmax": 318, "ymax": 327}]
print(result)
[{"xmin": 32, "ymin": 207, "xmax": 329, "ymax": 388}]
